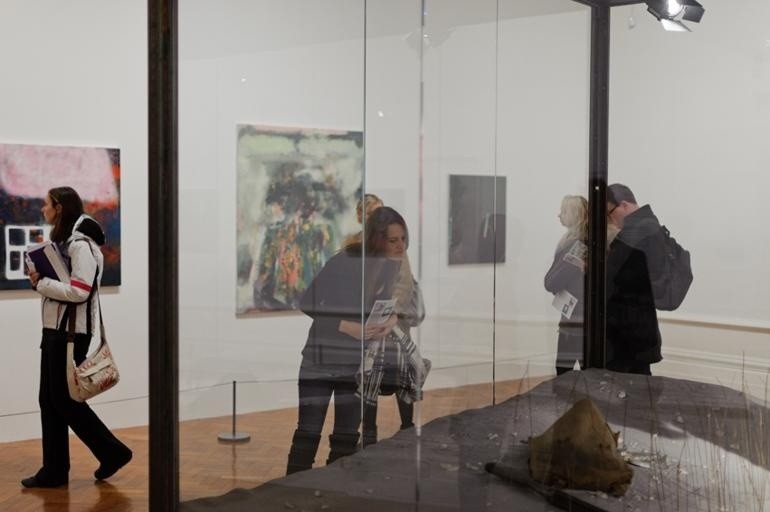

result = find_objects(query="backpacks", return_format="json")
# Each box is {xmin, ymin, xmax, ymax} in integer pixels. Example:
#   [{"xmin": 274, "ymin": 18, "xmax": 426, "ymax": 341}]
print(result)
[{"xmin": 641, "ymin": 214, "xmax": 693, "ymax": 311}]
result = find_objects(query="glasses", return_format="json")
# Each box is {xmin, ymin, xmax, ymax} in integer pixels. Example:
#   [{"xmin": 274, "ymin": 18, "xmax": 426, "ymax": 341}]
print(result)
[{"xmin": 606, "ymin": 204, "xmax": 619, "ymax": 217}]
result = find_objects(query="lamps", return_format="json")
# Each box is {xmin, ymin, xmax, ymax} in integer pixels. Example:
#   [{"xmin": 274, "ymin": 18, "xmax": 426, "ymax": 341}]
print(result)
[{"xmin": 644, "ymin": 0, "xmax": 705, "ymax": 33}]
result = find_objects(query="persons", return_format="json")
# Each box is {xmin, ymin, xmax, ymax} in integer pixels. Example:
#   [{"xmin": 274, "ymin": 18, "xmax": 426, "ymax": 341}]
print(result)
[
  {"xmin": 544, "ymin": 183, "xmax": 664, "ymax": 374},
  {"xmin": 287, "ymin": 193, "xmax": 426, "ymax": 474},
  {"xmin": 20, "ymin": 187, "xmax": 133, "ymax": 488}
]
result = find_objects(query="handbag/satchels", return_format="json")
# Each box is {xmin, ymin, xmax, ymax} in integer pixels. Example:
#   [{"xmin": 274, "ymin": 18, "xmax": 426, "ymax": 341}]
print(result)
[{"xmin": 66, "ymin": 342, "xmax": 120, "ymax": 403}]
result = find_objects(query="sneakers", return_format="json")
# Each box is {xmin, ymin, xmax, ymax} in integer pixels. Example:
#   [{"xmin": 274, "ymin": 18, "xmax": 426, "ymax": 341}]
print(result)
[
  {"xmin": 94, "ymin": 445, "xmax": 133, "ymax": 480},
  {"xmin": 21, "ymin": 469, "xmax": 69, "ymax": 488}
]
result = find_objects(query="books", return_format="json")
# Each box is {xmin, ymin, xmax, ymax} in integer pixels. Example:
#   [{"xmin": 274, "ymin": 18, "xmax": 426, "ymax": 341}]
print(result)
[{"xmin": 26, "ymin": 240, "xmax": 70, "ymax": 283}]
